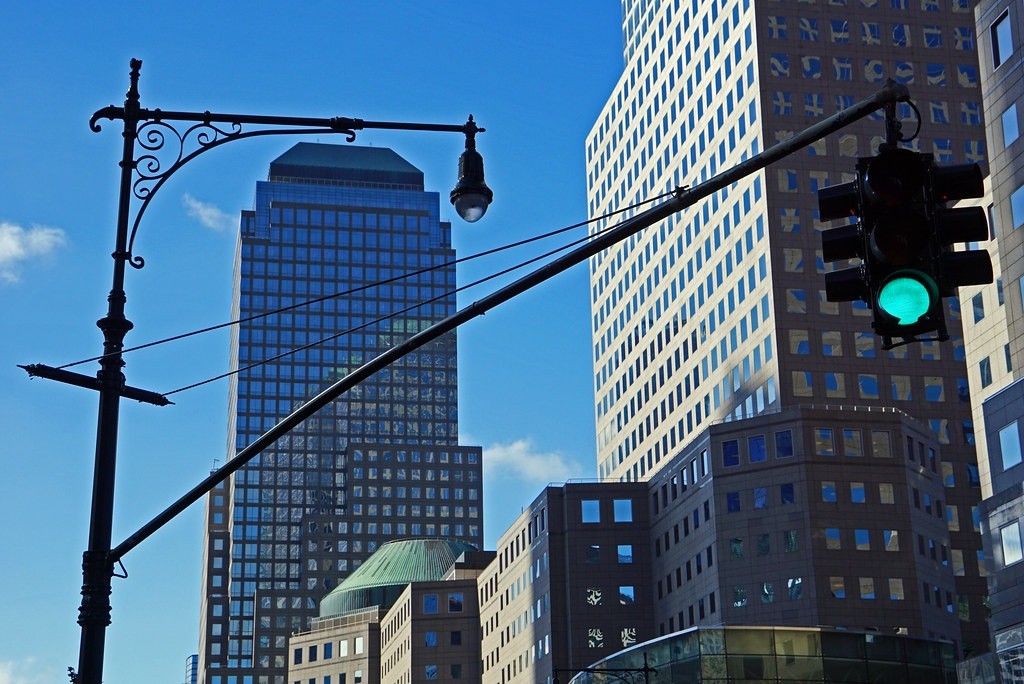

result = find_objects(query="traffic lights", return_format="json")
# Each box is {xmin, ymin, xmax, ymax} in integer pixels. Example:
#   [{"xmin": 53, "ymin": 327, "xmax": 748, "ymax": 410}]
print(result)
[
  {"xmin": 855, "ymin": 145, "xmax": 949, "ymax": 342},
  {"xmin": 815, "ymin": 157, "xmax": 876, "ymax": 313},
  {"xmin": 919, "ymin": 150, "xmax": 995, "ymax": 294}
]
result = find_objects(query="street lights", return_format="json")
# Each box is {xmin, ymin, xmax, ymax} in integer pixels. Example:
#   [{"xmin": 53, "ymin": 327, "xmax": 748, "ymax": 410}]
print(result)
[{"xmin": 63, "ymin": 61, "xmax": 491, "ymax": 684}]
